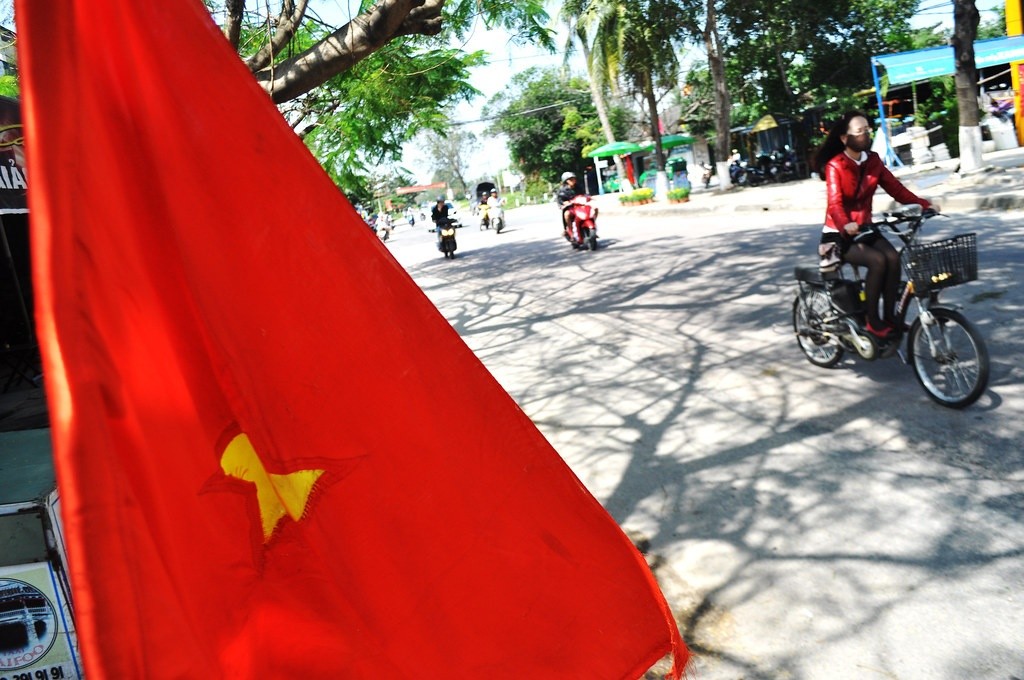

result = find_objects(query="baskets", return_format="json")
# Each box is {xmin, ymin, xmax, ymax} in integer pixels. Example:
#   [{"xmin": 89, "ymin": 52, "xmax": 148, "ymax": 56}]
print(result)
[{"xmin": 898, "ymin": 233, "xmax": 978, "ymax": 293}]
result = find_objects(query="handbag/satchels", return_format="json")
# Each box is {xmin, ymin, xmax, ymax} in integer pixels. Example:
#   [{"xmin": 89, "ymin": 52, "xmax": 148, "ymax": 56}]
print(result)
[{"xmin": 818, "ymin": 241, "xmax": 841, "ymax": 273}]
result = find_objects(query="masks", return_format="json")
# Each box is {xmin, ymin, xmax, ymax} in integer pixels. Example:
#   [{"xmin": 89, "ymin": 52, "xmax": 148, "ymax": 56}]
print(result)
[{"xmin": 845, "ymin": 133, "xmax": 872, "ymax": 152}]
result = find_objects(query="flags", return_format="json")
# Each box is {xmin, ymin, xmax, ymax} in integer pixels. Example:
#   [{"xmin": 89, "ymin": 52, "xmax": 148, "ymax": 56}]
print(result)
[{"xmin": 11, "ymin": 0, "xmax": 697, "ymax": 679}]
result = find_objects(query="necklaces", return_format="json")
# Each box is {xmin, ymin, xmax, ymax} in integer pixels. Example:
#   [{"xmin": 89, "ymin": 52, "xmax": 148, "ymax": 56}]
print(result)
[{"xmin": 478, "ymin": 188, "xmax": 506, "ymax": 218}]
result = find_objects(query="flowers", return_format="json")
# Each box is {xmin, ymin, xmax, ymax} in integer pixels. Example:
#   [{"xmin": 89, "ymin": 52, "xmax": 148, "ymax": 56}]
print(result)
[
  {"xmin": 619, "ymin": 188, "xmax": 656, "ymax": 202},
  {"xmin": 667, "ymin": 188, "xmax": 690, "ymax": 200}
]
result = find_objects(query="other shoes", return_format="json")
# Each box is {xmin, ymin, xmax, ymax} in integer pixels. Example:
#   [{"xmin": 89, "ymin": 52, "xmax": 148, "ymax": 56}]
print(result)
[
  {"xmin": 866, "ymin": 323, "xmax": 893, "ymax": 338},
  {"xmin": 885, "ymin": 319, "xmax": 913, "ymax": 331}
]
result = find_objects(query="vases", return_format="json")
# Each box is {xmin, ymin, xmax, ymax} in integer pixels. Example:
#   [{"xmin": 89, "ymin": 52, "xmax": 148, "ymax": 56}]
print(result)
[
  {"xmin": 620, "ymin": 199, "xmax": 655, "ymax": 206},
  {"xmin": 671, "ymin": 197, "xmax": 689, "ymax": 205}
]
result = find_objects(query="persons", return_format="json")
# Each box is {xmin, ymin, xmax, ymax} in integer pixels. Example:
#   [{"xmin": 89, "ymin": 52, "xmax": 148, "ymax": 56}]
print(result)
[
  {"xmin": 819, "ymin": 111, "xmax": 940, "ymax": 338},
  {"xmin": 431, "ymin": 198, "xmax": 449, "ymax": 241},
  {"xmin": 368, "ymin": 207, "xmax": 425, "ymax": 238},
  {"xmin": 557, "ymin": 171, "xmax": 598, "ymax": 243}
]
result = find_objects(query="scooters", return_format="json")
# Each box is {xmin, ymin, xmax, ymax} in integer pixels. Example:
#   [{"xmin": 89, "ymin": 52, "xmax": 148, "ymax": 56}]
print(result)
[
  {"xmin": 374, "ymin": 211, "xmax": 426, "ymax": 244},
  {"xmin": 476, "ymin": 197, "xmax": 508, "ymax": 235},
  {"xmin": 559, "ymin": 194, "xmax": 600, "ymax": 252},
  {"xmin": 790, "ymin": 199, "xmax": 991, "ymax": 408},
  {"xmin": 430, "ymin": 210, "xmax": 459, "ymax": 259}
]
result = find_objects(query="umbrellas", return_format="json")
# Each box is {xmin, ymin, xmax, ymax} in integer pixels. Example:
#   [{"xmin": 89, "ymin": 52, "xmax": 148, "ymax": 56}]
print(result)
[
  {"xmin": 588, "ymin": 141, "xmax": 642, "ymax": 193},
  {"xmin": 645, "ymin": 135, "xmax": 698, "ymax": 151}
]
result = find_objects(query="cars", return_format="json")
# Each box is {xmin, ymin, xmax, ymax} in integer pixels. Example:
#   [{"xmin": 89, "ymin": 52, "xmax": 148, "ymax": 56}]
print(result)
[{"xmin": 424, "ymin": 200, "xmax": 464, "ymax": 232}]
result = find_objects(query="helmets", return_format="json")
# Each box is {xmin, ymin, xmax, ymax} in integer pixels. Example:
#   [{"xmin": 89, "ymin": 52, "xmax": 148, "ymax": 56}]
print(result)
[
  {"xmin": 482, "ymin": 192, "xmax": 487, "ymax": 196},
  {"xmin": 490, "ymin": 188, "xmax": 497, "ymax": 193},
  {"xmin": 561, "ymin": 172, "xmax": 577, "ymax": 184},
  {"xmin": 437, "ymin": 197, "xmax": 444, "ymax": 203}
]
